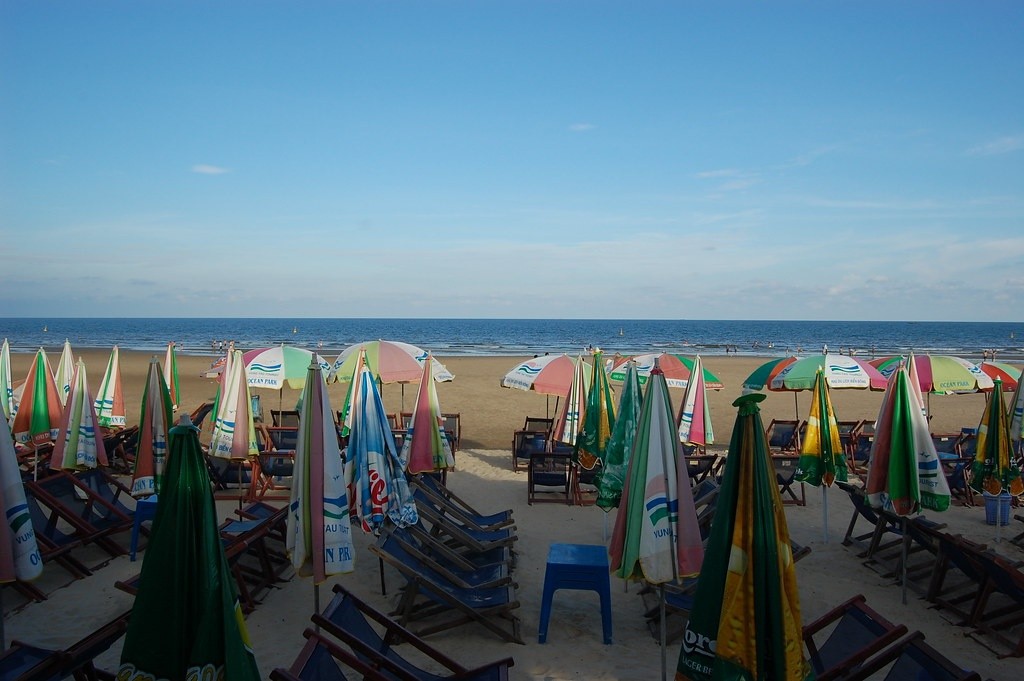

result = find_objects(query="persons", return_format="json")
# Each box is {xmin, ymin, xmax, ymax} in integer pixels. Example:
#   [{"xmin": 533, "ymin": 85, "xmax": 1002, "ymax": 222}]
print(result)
[
  {"xmin": 822, "ymin": 345, "xmax": 875, "ymax": 356},
  {"xmin": 992, "ymin": 351, "xmax": 995, "ymax": 362},
  {"xmin": 584, "ymin": 343, "xmax": 592, "ymax": 355},
  {"xmin": 726, "ymin": 341, "xmax": 799, "ymax": 354},
  {"xmin": 169, "ymin": 338, "xmax": 235, "ymax": 353},
  {"xmin": 983, "ymin": 350, "xmax": 987, "ymax": 361}
]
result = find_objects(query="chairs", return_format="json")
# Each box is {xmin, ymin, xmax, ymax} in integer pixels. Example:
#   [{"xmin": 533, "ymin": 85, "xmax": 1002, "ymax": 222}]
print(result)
[{"xmin": 0, "ymin": 403, "xmax": 1024, "ymax": 681}]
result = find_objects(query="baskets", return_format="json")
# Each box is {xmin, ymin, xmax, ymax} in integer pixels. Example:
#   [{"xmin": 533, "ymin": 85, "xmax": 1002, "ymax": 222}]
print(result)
[{"xmin": 984, "ymin": 490, "xmax": 1013, "ymax": 526}]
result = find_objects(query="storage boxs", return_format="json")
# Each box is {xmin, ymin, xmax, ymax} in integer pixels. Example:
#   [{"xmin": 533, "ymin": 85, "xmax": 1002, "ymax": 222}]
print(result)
[{"xmin": 983, "ymin": 490, "xmax": 1012, "ymax": 525}]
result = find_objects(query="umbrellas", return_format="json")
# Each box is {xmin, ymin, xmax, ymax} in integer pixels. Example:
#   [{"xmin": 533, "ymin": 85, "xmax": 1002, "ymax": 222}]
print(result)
[
  {"xmin": 741, "ymin": 349, "xmax": 889, "ymax": 544},
  {"xmin": 204, "ymin": 342, "xmax": 335, "ymax": 521},
  {"xmin": 286, "ymin": 352, "xmax": 357, "ymax": 633},
  {"xmin": 327, "ymin": 338, "xmax": 459, "ymax": 595},
  {"xmin": 0, "ymin": 398, "xmax": 44, "ymax": 653},
  {"xmin": 674, "ymin": 388, "xmax": 819, "ymax": 681},
  {"xmin": 0, "ymin": 337, "xmax": 180, "ymax": 521},
  {"xmin": 609, "ymin": 357, "xmax": 704, "ymax": 681},
  {"xmin": 863, "ymin": 357, "xmax": 951, "ymax": 604},
  {"xmin": 114, "ymin": 412, "xmax": 260, "ymax": 681},
  {"xmin": 500, "ymin": 352, "xmax": 724, "ymax": 541},
  {"xmin": 868, "ymin": 352, "xmax": 1024, "ymax": 544}
]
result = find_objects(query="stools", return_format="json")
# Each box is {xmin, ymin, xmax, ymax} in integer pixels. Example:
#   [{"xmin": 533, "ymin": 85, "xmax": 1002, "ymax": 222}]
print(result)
[
  {"xmin": 538, "ymin": 542, "xmax": 613, "ymax": 645},
  {"xmin": 131, "ymin": 494, "xmax": 158, "ymax": 563}
]
